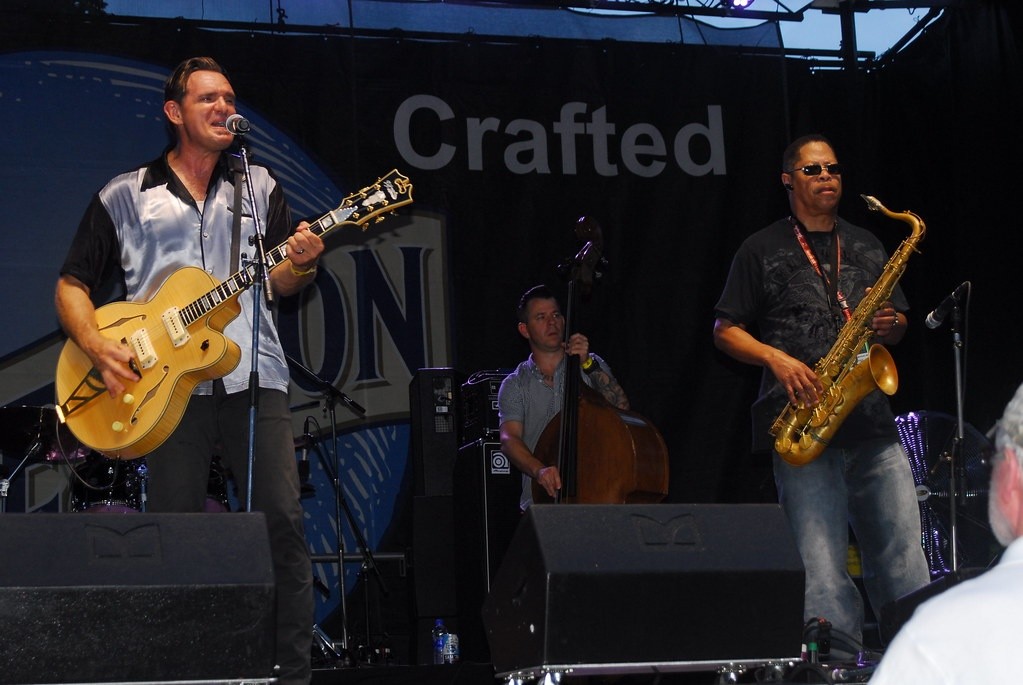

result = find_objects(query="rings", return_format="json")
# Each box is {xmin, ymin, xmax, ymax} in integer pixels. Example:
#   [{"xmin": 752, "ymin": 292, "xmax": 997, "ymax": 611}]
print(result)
[{"xmin": 298, "ymin": 249, "xmax": 304, "ymax": 254}]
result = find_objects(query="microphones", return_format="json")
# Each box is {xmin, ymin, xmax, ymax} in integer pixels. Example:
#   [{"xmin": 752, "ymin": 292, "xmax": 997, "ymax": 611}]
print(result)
[
  {"xmin": 225, "ymin": 114, "xmax": 252, "ymax": 135},
  {"xmin": 298, "ymin": 420, "xmax": 311, "ymax": 483},
  {"xmin": 925, "ymin": 281, "xmax": 969, "ymax": 330}
]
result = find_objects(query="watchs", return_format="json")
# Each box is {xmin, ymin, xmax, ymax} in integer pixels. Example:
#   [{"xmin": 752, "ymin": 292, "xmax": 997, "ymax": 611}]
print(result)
[{"xmin": 583, "ymin": 357, "xmax": 600, "ymax": 374}]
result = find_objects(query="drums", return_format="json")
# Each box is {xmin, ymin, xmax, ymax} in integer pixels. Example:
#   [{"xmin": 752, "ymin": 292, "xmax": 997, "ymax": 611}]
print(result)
[
  {"xmin": 206, "ymin": 454, "xmax": 229, "ymax": 513},
  {"xmin": 70, "ymin": 451, "xmax": 148, "ymax": 513}
]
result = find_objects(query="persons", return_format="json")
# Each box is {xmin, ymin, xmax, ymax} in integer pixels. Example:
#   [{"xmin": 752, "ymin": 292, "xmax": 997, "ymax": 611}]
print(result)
[
  {"xmin": 712, "ymin": 133, "xmax": 928, "ymax": 655},
  {"xmin": 497, "ymin": 285, "xmax": 633, "ymax": 510},
  {"xmin": 864, "ymin": 384, "xmax": 1023, "ymax": 685},
  {"xmin": 54, "ymin": 54, "xmax": 325, "ymax": 685}
]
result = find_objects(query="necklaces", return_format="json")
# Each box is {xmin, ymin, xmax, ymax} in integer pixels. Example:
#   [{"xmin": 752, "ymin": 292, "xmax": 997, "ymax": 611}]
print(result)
[{"xmin": 542, "ymin": 374, "xmax": 554, "ymax": 381}]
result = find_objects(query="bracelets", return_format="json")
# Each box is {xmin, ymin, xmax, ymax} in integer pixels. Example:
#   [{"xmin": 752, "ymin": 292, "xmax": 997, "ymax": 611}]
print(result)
[
  {"xmin": 891, "ymin": 312, "xmax": 899, "ymax": 329},
  {"xmin": 581, "ymin": 357, "xmax": 593, "ymax": 369}
]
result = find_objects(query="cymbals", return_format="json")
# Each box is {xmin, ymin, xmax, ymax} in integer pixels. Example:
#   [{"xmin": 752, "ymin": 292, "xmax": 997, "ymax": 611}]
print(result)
[{"xmin": 0, "ymin": 405, "xmax": 92, "ymax": 465}]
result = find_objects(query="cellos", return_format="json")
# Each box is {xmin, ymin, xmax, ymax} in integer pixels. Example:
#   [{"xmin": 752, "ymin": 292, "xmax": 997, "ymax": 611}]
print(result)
[{"xmin": 532, "ymin": 214, "xmax": 670, "ymax": 505}]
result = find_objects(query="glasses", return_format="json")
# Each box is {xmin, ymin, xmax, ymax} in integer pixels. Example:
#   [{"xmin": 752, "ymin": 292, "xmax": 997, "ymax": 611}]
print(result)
[{"xmin": 787, "ymin": 164, "xmax": 843, "ymax": 176}]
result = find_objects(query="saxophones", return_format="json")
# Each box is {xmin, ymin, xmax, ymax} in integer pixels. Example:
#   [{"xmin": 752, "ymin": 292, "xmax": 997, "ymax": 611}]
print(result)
[{"xmin": 769, "ymin": 194, "xmax": 927, "ymax": 467}]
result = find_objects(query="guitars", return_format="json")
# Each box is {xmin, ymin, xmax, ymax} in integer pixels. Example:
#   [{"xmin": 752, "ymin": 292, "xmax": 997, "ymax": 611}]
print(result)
[{"xmin": 55, "ymin": 168, "xmax": 414, "ymax": 462}]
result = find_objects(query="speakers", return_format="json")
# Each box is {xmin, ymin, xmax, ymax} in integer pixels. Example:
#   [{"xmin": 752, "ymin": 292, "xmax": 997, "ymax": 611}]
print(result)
[
  {"xmin": 879, "ymin": 564, "xmax": 990, "ymax": 653},
  {"xmin": 450, "ymin": 437, "xmax": 804, "ymax": 682},
  {"xmin": 0, "ymin": 514, "xmax": 277, "ymax": 685}
]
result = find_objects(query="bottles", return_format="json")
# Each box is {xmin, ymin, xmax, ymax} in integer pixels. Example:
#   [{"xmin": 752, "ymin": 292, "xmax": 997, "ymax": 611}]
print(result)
[
  {"xmin": 805, "ymin": 642, "xmax": 821, "ymax": 664},
  {"xmin": 431, "ymin": 619, "xmax": 448, "ymax": 665}
]
